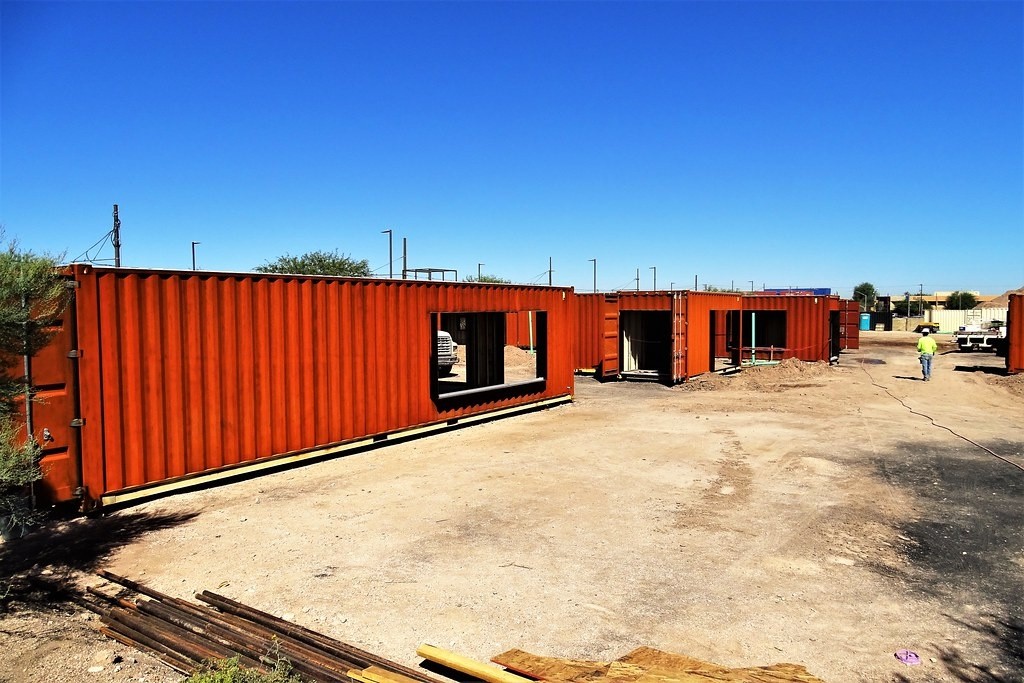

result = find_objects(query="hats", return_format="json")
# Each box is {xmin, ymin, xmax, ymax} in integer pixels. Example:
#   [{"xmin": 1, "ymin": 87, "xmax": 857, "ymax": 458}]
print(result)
[{"xmin": 921, "ymin": 328, "xmax": 930, "ymax": 333}]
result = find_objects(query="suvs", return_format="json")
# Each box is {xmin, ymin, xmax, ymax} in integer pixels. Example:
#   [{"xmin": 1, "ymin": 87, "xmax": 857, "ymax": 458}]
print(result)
[{"xmin": 438, "ymin": 330, "xmax": 459, "ymax": 377}]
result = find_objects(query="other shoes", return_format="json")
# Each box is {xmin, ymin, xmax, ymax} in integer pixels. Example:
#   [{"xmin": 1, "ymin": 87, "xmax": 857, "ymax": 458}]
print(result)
[{"xmin": 923, "ymin": 376, "xmax": 931, "ymax": 381}]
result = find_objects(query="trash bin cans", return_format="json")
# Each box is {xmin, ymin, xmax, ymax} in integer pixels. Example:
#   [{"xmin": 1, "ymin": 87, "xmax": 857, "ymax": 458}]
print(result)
[{"xmin": 859, "ymin": 313, "xmax": 870, "ymax": 331}]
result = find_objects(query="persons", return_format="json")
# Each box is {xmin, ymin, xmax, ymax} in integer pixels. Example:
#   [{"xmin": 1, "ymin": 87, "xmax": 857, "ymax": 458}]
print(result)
[{"xmin": 917, "ymin": 328, "xmax": 937, "ymax": 382}]
